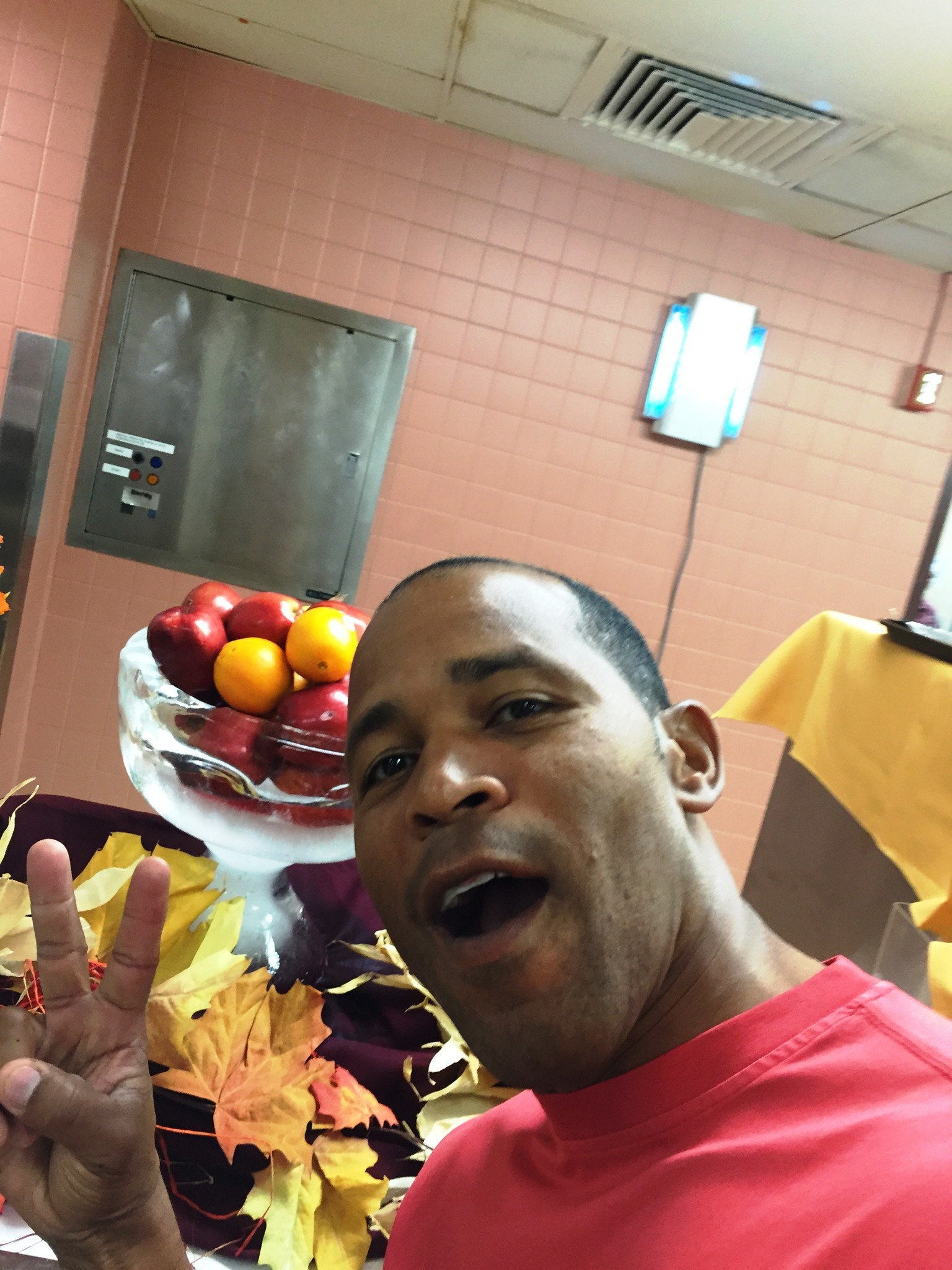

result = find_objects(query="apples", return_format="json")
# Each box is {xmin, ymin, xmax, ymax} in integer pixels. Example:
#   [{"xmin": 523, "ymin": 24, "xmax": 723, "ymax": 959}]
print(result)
[{"xmin": 148, "ymin": 580, "xmax": 372, "ymax": 824}]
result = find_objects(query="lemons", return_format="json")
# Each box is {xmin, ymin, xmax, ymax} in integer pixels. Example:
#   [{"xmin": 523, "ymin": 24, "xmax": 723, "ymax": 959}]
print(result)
[
  {"xmin": 214, "ymin": 637, "xmax": 294, "ymax": 715},
  {"xmin": 286, "ymin": 606, "xmax": 358, "ymax": 683},
  {"xmin": 293, "ymin": 670, "xmax": 316, "ymax": 691}
]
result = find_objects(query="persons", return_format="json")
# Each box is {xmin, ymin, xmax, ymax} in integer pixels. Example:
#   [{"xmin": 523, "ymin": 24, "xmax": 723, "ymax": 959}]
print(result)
[{"xmin": 0, "ymin": 556, "xmax": 952, "ymax": 1270}]
